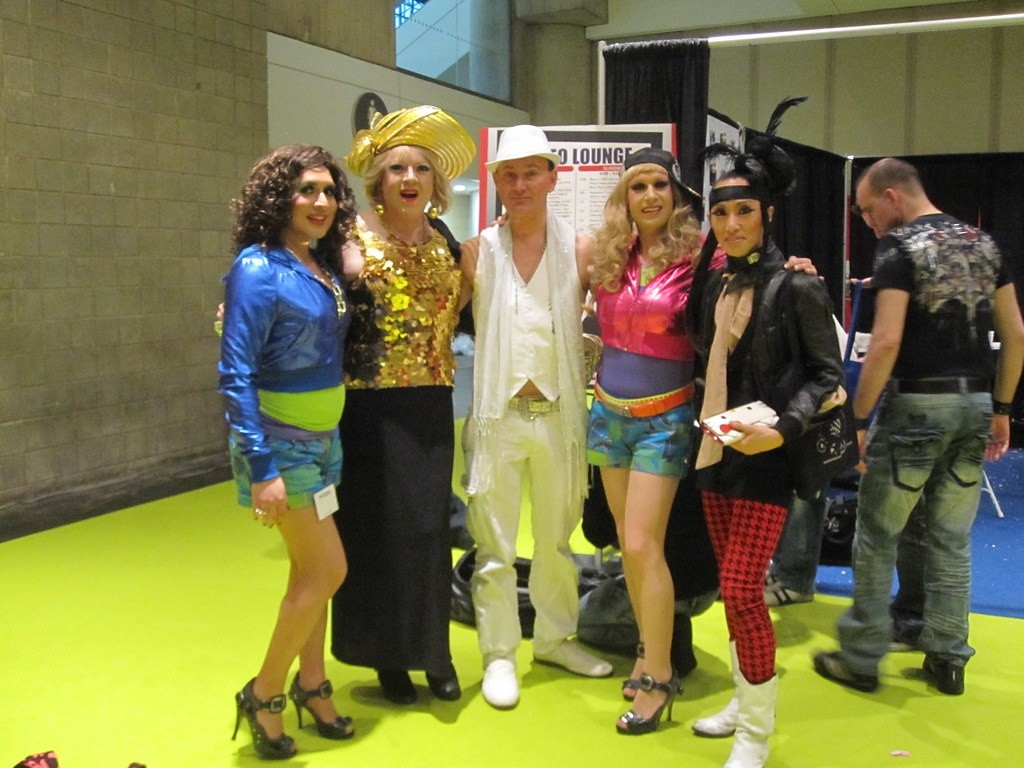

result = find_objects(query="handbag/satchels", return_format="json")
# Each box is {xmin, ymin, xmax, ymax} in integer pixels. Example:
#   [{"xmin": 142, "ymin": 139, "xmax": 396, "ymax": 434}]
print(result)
[{"xmin": 778, "ymin": 267, "xmax": 860, "ymax": 501}]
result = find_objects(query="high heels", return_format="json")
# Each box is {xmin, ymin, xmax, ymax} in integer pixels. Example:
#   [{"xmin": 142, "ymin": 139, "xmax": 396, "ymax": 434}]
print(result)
[
  {"xmin": 287, "ymin": 670, "xmax": 355, "ymax": 742},
  {"xmin": 621, "ymin": 642, "xmax": 684, "ymax": 701},
  {"xmin": 231, "ymin": 677, "xmax": 298, "ymax": 760},
  {"xmin": 616, "ymin": 666, "xmax": 684, "ymax": 734}
]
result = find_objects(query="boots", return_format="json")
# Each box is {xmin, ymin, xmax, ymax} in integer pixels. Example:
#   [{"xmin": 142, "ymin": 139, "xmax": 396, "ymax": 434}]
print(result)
[
  {"xmin": 691, "ymin": 683, "xmax": 741, "ymax": 738},
  {"xmin": 722, "ymin": 638, "xmax": 778, "ymax": 768}
]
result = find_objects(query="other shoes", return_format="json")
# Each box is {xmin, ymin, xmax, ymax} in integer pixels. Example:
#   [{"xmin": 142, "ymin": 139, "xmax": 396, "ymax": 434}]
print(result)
[
  {"xmin": 920, "ymin": 653, "xmax": 965, "ymax": 695},
  {"xmin": 813, "ymin": 651, "xmax": 878, "ymax": 692},
  {"xmin": 533, "ymin": 639, "xmax": 614, "ymax": 678},
  {"xmin": 761, "ymin": 584, "xmax": 815, "ymax": 607},
  {"xmin": 480, "ymin": 658, "xmax": 520, "ymax": 710},
  {"xmin": 762, "ymin": 572, "xmax": 782, "ymax": 586}
]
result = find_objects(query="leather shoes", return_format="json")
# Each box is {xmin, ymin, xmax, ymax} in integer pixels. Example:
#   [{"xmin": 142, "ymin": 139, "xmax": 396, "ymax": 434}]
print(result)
[
  {"xmin": 424, "ymin": 663, "xmax": 461, "ymax": 701},
  {"xmin": 376, "ymin": 669, "xmax": 418, "ymax": 704}
]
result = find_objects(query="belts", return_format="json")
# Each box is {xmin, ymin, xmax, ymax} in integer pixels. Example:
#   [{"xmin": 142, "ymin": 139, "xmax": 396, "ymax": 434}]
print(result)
[
  {"xmin": 592, "ymin": 366, "xmax": 697, "ymax": 419},
  {"xmin": 885, "ymin": 376, "xmax": 992, "ymax": 395},
  {"xmin": 506, "ymin": 393, "xmax": 561, "ymax": 422}
]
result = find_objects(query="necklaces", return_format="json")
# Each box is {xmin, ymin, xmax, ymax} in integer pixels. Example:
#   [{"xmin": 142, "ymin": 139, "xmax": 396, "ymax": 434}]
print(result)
[
  {"xmin": 378, "ymin": 216, "xmax": 426, "ymax": 248},
  {"xmin": 285, "ymin": 248, "xmax": 346, "ymax": 317}
]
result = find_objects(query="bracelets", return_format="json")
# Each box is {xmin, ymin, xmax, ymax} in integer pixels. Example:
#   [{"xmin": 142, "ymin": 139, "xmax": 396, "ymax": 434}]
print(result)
[
  {"xmin": 854, "ymin": 418, "xmax": 868, "ymax": 431},
  {"xmin": 992, "ymin": 399, "xmax": 1012, "ymax": 416}
]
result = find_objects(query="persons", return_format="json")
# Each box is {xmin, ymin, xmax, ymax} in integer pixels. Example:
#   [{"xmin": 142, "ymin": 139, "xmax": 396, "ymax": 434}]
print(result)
[
  {"xmin": 216, "ymin": 144, "xmax": 356, "ymax": 760},
  {"xmin": 462, "ymin": 125, "xmax": 612, "ymax": 706},
  {"xmin": 814, "ymin": 158, "xmax": 1024, "ymax": 696},
  {"xmin": 216, "ymin": 105, "xmax": 477, "ymax": 704},
  {"xmin": 686, "ymin": 137, "xmax": 845, "ymax": 768},
  {"xmin": 493, "ymin": 147, "xmax": 825, "ymax": 735}
]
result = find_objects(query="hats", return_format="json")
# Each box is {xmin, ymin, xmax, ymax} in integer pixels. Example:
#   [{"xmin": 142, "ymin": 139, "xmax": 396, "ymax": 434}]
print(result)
[
  {"xmin": 343, "ymin": 104, "xmax": 478, "ymax": 184},
  {"xmin": 624, "ymin": 148, "xmax": 703, "ymax": 199},
  {"xmin": 483, "ymin": 125, "xmax": 561, "ymax": 175}
]
